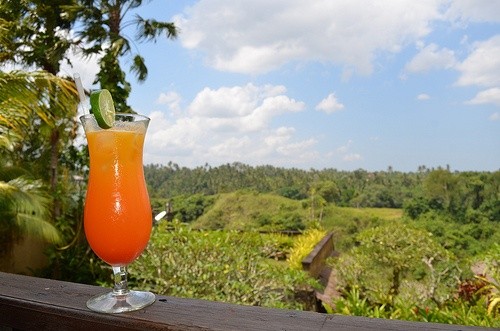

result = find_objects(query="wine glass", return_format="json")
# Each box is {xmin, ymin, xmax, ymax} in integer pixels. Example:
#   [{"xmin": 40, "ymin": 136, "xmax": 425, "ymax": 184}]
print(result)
[{"xmin": 79, "ymin": 112, "xmax": 156, "ymax": 314}]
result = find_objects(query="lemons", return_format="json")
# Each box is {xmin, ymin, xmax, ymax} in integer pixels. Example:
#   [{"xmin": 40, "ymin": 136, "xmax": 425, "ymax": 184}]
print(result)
[{"xmin": 90, "ymin": 88, "xmax": 116, "ymax": 130}]
